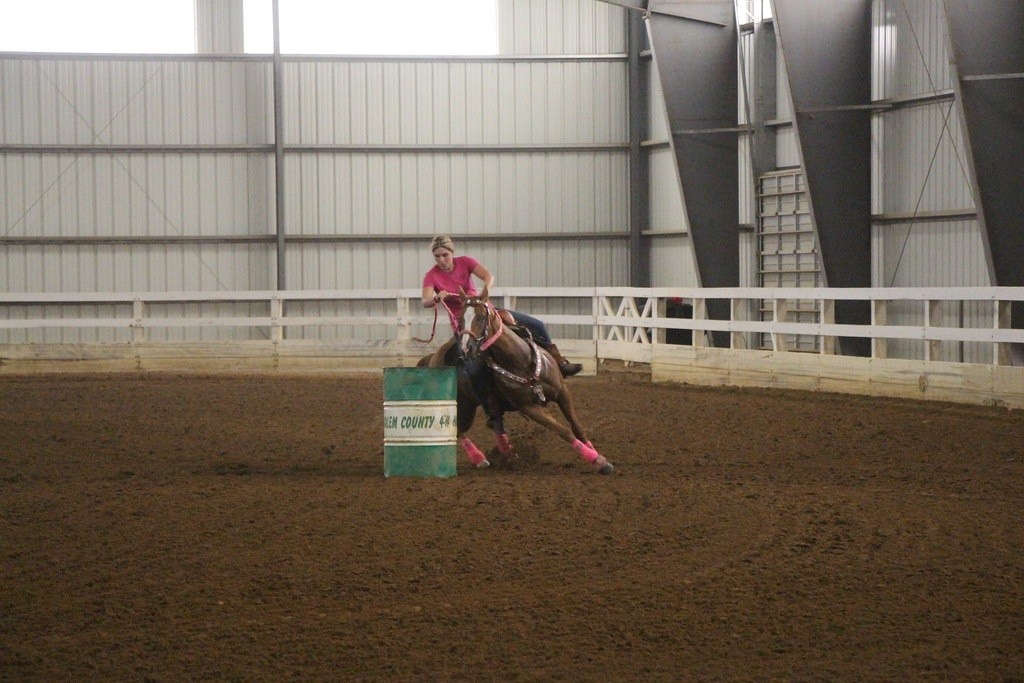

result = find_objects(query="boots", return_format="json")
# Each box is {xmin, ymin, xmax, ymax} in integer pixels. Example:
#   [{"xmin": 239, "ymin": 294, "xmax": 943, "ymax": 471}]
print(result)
[{"xmin": 546, "ymin": 344, "xmax": 582, "ymax": 379}]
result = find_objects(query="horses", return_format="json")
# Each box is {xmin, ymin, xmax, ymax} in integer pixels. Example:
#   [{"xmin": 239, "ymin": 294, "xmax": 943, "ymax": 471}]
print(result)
[{"xmin": 414, "ymin": 283, "xmax": 615, "ymax": 476}]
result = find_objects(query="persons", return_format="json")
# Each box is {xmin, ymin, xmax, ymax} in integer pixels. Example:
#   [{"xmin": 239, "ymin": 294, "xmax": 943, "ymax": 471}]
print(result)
[{"xmin": 422, "ymin": 235, "xmax": 583, "ymax": 430}]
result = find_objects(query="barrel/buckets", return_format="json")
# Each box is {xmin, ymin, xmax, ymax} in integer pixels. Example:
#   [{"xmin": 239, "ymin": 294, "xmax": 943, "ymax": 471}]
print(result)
[{"xmin": 383, "ymin": 366, "xmax": 458, "ymax": 478}]
